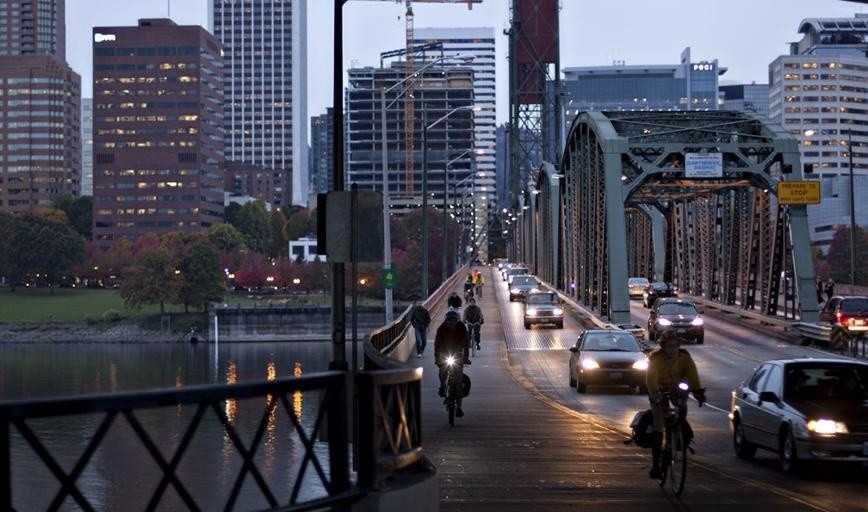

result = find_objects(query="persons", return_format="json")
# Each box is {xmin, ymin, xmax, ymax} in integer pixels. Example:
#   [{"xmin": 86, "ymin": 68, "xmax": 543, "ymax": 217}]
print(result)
[
  {"xmin": 644, "ymin": 330, "xmax": 706, "ymax": 480},
  {"xmin": 410, "ymin": 299, "xmax": 431, "ymax": 358},
  {"xmin": 434, "ymin": 311, "xmax": 470, "ymax": 418},
  {"xmin": 463, "ymin": 297, "xmax": 484, "ymax": 351},
  {"xmin": 447, "ymin": 269, "xmax": 485, "ymax": 320},
  {"xmin": 815, "ymin": 275, "xmax": 835, "ymax": 304}
]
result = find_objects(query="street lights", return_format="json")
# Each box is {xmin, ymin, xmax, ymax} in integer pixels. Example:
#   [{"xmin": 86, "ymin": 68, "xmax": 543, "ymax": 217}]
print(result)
[
  {"xmin": 451, "ymin": 171, "xmax": 487, "ymax": 274},
  {"xmin": 294, "ymin": 278, "xmax": 300, "ymax": 296},
  {"xmin": 440, "ymin": 149, "xmax": 485, "ymax": 283},
  {"xmin": 804, "ymin": 127, "xmax": 857, "ymax": 287},
  {"xmin": 380, "ymin": 55, "xmax": 476, "ymax": 324},
  {"xmin": 358, "ymin": 279, "xmax": 365, "ymax": 306},
  {"xmin": 228, "ymin": 274, "xmax": 235, "ymax": 296},
  {"xmin": 94, "ymin": 266, "xmax": 99, "ymax": 288},
  {"xmin": 267, "ymin": 276, "xmax": 274, "ymax": 298},
  {"xmin": 422, "ymin": 104, "xmax": 481, "ymax": 302}
]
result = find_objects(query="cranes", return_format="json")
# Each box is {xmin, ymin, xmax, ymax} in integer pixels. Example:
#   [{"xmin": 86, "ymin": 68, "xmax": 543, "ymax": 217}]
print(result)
[{"xmin": 395, "ymin": 1, "xmax": 485, "ymax": 195}]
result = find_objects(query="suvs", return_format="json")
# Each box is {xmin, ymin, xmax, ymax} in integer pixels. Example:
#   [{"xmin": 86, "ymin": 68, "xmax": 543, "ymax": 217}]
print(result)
[
  {"xmin": 522, "ymin": 289, "xmax": 565, "ymax": 329},
  {"xmin": 648, "ymin": 296, "xmax": 704, "ymax": 344}
]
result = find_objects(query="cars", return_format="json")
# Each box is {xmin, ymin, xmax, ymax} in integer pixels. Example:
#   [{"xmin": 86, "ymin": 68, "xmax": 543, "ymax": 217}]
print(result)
[
  {"xmin": 492, "ymin": 258, "xmax": 544, "ymax": 302},
  {"xmin": 628, "ymin": 277, "xmax": 650, "ymax": 297},
  {"xmin": 728, "ymin": 357, "xmax": 868, "ymax": 472},
  {"xmin": 568, "ymin": 329, "xmax": 650, "ymax": 395},
  {"xmin": 3, "ymin": 275, "xmax": 123, "ymax": 290},
  {"xmin": 435, "ymin": 351, "xmax": 472, "ymax": 428},
  {"xmin": 642, "ymin": 281, "xmax": 678, "ymax": 308},
  {"xmin": 777, "ymin": 270, "xmax": 792, "ymax": 294},
  {"xmin": 232, "ymin": 285, "xmax": 331, "ymax": 295}
]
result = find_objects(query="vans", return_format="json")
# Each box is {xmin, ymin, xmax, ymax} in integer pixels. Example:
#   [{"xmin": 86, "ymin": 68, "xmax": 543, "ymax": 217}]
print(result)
[{"xmin": 820, "ymin": 294, "xmax": 868, "ymax": 339}]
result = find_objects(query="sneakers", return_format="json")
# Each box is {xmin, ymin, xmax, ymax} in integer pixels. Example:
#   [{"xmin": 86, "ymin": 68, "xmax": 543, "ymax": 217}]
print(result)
[
  {"xmin": 649, "ymin": 468, "xmax": 662, "ymax": 479},
  {"xmin": 455, "ymin": 408, "xmax": 464, "ymax": 417},
  {"xmin": 417, "ymin": 352, "xmax": 423, "ymax": 358},
  {"xmin": 438, "ymin": 384, "xmax": 445, "ymax": 396}
]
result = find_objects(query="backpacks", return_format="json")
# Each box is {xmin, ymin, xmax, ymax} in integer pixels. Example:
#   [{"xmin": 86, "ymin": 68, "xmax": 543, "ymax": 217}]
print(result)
[{"xmin": 623, "ymin": 406, "xmax": 655, "ymax": 450}]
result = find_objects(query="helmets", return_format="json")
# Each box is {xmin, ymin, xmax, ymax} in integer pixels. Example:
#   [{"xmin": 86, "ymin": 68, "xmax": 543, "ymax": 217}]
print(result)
[
  {"xmin": 659, "ymin": 328, "xmax": 682, "ymax": 344},
  {"xmin": 445, "ymin": 311, "xmax": 459, "ymax": 320}
]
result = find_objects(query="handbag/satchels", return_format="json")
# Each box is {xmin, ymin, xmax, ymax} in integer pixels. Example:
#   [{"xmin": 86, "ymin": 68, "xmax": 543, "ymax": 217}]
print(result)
[{"xmin": 462, "ymin": 373, "xmax": 472, "ymax": 396}]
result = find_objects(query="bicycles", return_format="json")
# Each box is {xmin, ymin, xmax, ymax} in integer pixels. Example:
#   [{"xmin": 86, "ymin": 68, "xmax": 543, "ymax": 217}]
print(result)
[
  {"xmin": 653, "ymin": 383, "xmax": 706, "ymax": 496},
  {"xmin": 475, "ymin": 283, "xmax": 484, "ymax": 301},
  {"xmin": 464, "ymin": 320, "xmax": 481, "ymax": 358},
  {"xmin": 463, "ymin": 284, "xmax": 475, "ymax": 303},
  {"xmin": 448, "ymin": 305, "xmax": 462, "ymax": 317}
]
